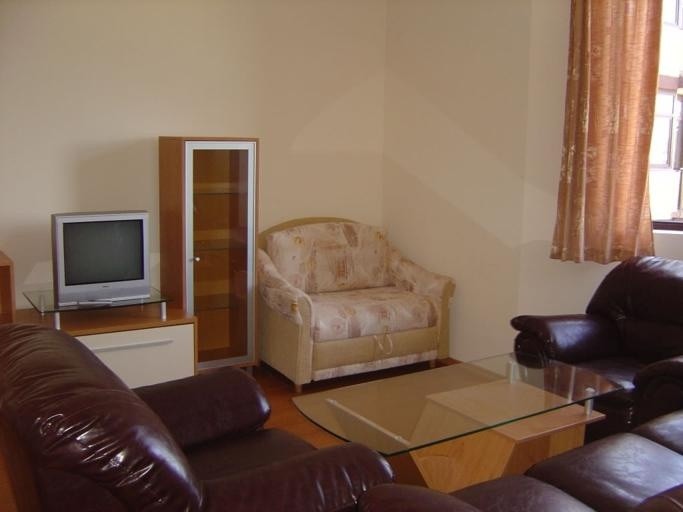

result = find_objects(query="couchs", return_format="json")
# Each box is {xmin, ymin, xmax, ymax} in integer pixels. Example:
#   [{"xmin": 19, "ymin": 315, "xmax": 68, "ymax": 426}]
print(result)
[
  {"xmin": 357, "ymin": 407, "xmax": 683, "ymax": 512},
  {"xmin": 258, "ymin": 217, "xmax": 456, "ymax": 394},
  {"xmin": 510, "ymin": 256, "xmax": 683, "ymax": 441},
  {"xmin": 0, "ymin": 322, "xmax": 396, "ymax": 512}
]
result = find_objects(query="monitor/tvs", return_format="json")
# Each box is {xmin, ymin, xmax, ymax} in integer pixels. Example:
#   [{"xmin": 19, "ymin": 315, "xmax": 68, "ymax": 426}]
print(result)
[{"xmin": 51, "ymin": 210, "xmax": 151, "ymax": 306}]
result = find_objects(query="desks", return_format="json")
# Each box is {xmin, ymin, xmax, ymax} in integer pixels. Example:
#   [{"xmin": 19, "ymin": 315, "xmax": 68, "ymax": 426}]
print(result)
[{"xmin": 23, "ymin": 289, "xmax": 167, "ymax": 331}]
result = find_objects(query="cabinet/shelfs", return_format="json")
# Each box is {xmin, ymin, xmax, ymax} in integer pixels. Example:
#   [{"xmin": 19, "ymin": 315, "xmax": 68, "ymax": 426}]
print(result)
[
  {"xmin": 15, "ymin": 303, "xmax": 198, "ymax": 391},
  {"xmin": 159, "ymin": 136, "xmax": 260, "ymax": 376}
]
